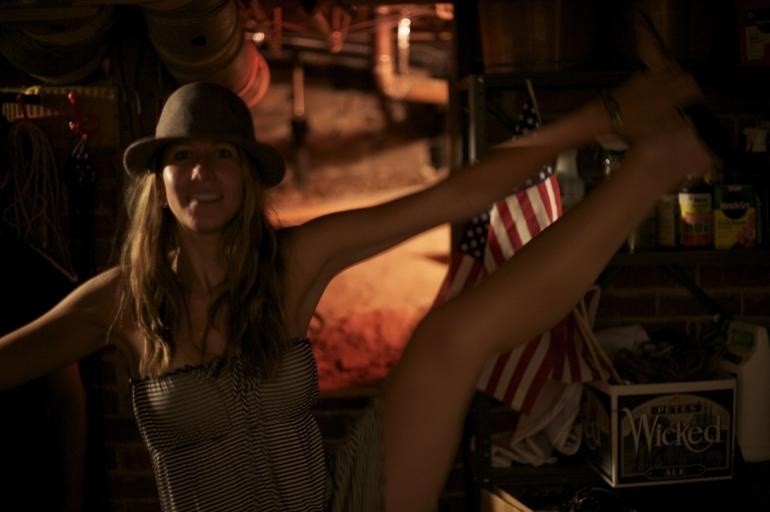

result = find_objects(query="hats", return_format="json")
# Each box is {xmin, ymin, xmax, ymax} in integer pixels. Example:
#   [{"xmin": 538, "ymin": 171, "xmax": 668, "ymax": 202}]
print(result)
[{"xmin": 122, "ymin": 80, "xmax": 287, "ymax": 190}]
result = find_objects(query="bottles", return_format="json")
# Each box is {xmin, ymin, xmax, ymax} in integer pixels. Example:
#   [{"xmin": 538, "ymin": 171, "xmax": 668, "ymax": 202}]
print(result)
[{"xmin": 679, "ymin": 171, "xmax": 747, "ymax": 251}]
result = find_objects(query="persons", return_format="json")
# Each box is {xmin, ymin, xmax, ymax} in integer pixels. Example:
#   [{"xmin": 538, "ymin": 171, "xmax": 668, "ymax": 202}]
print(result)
[{"xmin": 0, "ymin": 26, "xmax": 713, "ymax": 511}]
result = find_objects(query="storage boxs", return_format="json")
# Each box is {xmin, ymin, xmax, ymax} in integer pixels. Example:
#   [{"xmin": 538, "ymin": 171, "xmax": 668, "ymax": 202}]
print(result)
[{"xmin": 583, "ymin": 371, "xmax": 737, "ymax": 489}]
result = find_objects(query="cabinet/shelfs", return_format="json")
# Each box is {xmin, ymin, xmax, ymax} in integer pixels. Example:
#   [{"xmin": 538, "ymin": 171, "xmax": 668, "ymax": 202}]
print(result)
[{"xmin": 450, "ymin": 74, "xmax": 769, "ymax": 512}]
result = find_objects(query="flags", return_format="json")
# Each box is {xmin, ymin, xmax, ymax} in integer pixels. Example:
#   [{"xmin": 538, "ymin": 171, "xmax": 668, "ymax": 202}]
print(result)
[
  {"xmin": 428, "ymin": 190, "xmax": 554, "ymax": 422},
  {"xmin": 481, "ymin": 97, "xmax": 615, "ymax": 386}
]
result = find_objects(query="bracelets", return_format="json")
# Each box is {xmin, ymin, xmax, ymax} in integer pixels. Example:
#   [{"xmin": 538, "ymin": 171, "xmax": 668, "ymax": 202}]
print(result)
[{"xmin": 598, "ymin": 89, "xmax": 624, "ymax": 130}]
